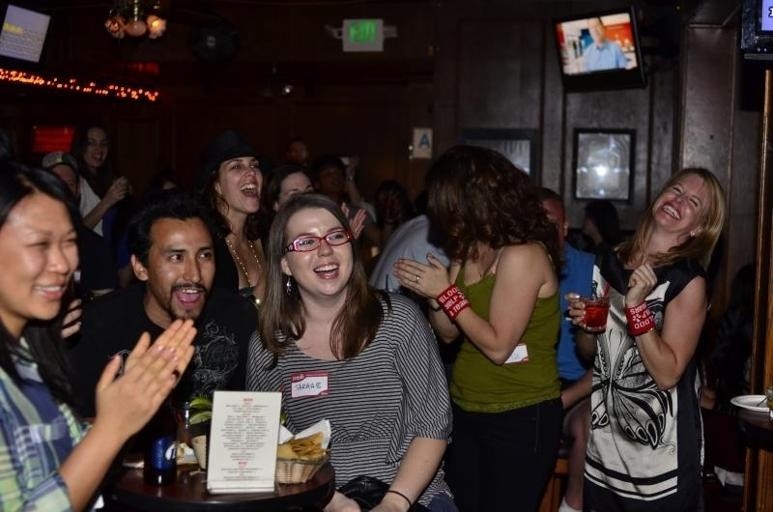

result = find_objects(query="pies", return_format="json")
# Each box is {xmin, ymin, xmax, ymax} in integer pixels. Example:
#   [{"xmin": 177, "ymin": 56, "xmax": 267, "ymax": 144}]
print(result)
[{"xmin": 276, "ymin": 432, "xmax": 324, "ymax": 461}]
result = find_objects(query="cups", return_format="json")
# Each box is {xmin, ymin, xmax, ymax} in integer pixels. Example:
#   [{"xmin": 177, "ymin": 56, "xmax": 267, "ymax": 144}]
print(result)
[
  {"xmin": 193, "ymin": 436, "xmax": 208, "ymax": 469},
  {"xmin": 580, "ymin": 297, "xmax": 609, "ymax": 332}
]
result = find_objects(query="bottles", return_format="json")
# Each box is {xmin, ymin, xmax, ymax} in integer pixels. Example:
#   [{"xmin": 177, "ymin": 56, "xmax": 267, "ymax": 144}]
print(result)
[{"xmin": 144, "ymin": 412, "xmax": 178, "ymax": 487}]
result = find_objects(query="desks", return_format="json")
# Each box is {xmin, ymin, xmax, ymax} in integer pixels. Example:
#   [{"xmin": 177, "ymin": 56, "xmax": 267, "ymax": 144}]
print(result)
[{"xmin": 108, "ymin": 436, "xmax": 336, "ymax": 512}]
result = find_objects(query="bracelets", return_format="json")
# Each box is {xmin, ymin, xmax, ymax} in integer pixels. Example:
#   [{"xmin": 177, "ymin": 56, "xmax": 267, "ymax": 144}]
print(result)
[
  {"xmin": 386, "ymin": 490, "xmax": 412, "ymax": 507},
  {"xmin": 247, "ymin": 294, "xmax": 262, "ymax": 309}
]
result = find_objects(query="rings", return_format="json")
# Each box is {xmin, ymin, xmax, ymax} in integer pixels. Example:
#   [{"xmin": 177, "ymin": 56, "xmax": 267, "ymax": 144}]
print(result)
[{"xmin": 416, "ymin": 275, "xmax": 419, "ymax": 283}]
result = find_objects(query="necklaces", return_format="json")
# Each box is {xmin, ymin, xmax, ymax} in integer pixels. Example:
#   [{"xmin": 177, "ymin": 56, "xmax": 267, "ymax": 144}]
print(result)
[
  {"xmin": 225, "ymin": 238, "xmax": 262, "ymax": 287},
  {"xmin": 477, "ymin": 250, "xmax": 497, "ymax": 277}
]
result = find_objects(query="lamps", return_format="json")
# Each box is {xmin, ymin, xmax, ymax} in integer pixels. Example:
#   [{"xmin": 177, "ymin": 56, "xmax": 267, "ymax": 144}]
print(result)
[{"xmin": 103, "ymin": 1, "xmax": 167, "ymax": 40}]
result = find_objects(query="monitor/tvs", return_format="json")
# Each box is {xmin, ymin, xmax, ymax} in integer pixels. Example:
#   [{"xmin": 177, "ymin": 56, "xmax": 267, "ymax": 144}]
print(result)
[{"xmin": 553, "ymin": 8, "xmax": 645, "ymax": 95}]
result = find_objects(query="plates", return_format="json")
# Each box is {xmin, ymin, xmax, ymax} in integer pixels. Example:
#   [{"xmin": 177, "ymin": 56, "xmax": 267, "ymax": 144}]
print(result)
[{"xmin": 731, "ymin": 394, "xmax": 769, "ymax": 412}]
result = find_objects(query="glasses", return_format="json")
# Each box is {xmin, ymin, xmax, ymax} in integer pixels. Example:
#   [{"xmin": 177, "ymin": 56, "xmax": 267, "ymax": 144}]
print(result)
[{"xmin": 281, "ymin": 229, "xmax": 351, "ymax": 253}]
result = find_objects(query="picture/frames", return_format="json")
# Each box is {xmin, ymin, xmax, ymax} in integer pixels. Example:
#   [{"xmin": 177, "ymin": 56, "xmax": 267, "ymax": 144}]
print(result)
[
  {"xmin": 461, "ymin": 127, "xmax": 541, "ymax": 189},
  {"xmin": 571, "ymin": 128, "xmax": 638, "ymax": 206}
]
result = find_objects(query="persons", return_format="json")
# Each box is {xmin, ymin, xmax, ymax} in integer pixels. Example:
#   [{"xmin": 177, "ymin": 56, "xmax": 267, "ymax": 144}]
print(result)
[
  {"xmin": 77, "ymin": 126, "xmax": 133, "ymax": 231},
  {"xmin": 392, "ymin": 145, "xmax": 562, "ymax": 512},
  {"xmin": 310, "ymin": 154, "xmax": 377, "ymax": 225},
  {"xmin": 65, "ymin": 203, "xmax": 245, "ymax": 457},
  {"xmin": 0, "ymin": 163, "xmax": 197, "ymax": 511},
  {"xmin": 534, "ymin": 186, "xmax": 601, "ymax": 511},
  {"xmin": 368, "ymin": 215, "xmax": 451, "ymax": 294},
  {"xmin": 262, "ymin": 165, "xmax": 365, "ymax": 260},
  {"xmin": 584, "ymin": 16, "xmax": 628, "ymax": 73},
  {"xmin": 375, "ymin": 178, "xmax": 411, "ymax": 227},
  {"xmin": 565, "ymin": 168, "xmax": 726, "ymax": 511},
  {"xmin": 119, "ymin": 172, "xmax": 179, "ymax": 288},
  {"xmin": 46, "ymin": 163, "xmax": 77, "ymax": 197},
  {"xmin": 193, "ymin": 128, "xmax": 263, "ymax": 310},
  {"xmin": 245, "ymin": 192, "xmax": 457, "ymax": 511}
]
